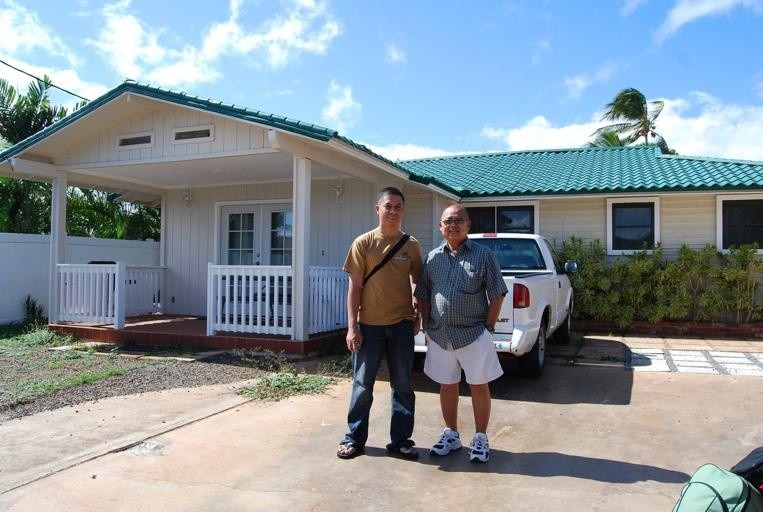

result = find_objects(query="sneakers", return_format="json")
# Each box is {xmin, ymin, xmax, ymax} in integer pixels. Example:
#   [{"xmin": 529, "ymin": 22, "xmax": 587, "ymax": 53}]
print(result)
[
  {"xmin": 470, "ymin": 432, "xmax": 489, "ymax": 463},
  {"xmin": 430, "ymin": 428, "xmax": 461, "ymax": 456}
]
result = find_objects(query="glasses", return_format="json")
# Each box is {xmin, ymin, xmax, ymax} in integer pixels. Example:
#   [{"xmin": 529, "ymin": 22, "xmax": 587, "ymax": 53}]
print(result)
[{"xmin": 443, "ymin": 219, "xmax": 464, "ymax": 224}]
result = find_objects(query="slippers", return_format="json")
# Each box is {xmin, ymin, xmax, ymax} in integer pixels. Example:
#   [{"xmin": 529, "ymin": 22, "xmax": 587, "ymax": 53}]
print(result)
[
  {"xmin": 337, "ymin": 443, "xmax": 364, "ymax": 458},
  {"xmin": 386, "ymin": 440, "xmax": 419, "ymax": 459}
]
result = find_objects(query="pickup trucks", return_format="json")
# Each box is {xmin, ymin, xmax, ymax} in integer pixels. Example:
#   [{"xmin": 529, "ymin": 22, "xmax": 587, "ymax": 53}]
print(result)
[{"xmin": 409, "ymin": 231, "xmax": 578, "ymax": 380}]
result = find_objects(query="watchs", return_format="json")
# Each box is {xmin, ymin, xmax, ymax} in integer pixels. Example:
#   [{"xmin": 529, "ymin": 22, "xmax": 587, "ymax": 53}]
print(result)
[{"xmin": 486, "ymin": 325, "xmax": 495, "ymax": 332}]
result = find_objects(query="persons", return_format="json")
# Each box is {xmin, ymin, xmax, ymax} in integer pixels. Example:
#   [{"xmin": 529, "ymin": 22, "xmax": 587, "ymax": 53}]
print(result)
[
  {"xmin": 337, "ymin": 187, "xmax": 423, "ymax": 459},
  {"xmin": 413, "ymin": 203, "xmax": 508, "ymax": 463}
]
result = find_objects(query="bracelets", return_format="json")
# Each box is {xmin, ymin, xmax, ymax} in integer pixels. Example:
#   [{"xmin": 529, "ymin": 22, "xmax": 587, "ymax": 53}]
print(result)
[{"xmin": 416, "ymin": 316, "xmax": 421, "ymax": 320}]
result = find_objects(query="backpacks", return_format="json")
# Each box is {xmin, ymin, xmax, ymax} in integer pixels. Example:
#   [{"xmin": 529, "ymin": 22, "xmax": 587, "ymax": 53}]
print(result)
[{"xmin": 672, "ymin": 447, "xmax": 763, "ymax": 512}]
